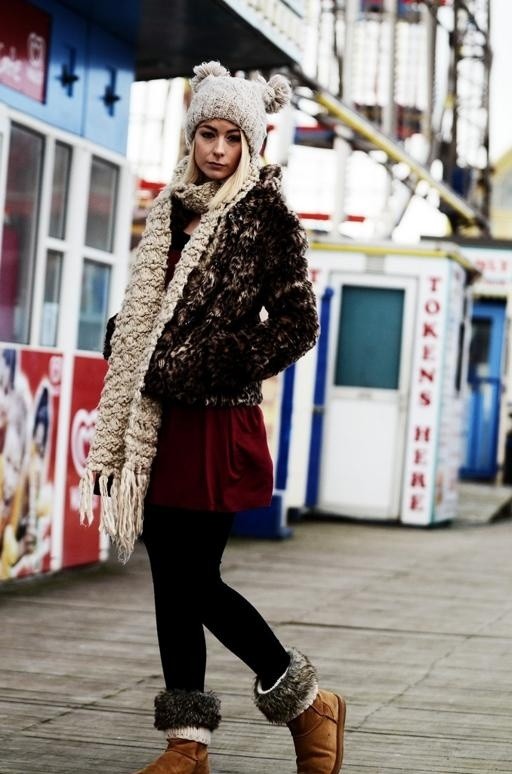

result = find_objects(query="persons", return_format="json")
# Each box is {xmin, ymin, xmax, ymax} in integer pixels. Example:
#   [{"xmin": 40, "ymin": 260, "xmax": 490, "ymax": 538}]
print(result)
[{"xmin": 75, "ymin": 54, "xmax": 352, "ymax": 772}]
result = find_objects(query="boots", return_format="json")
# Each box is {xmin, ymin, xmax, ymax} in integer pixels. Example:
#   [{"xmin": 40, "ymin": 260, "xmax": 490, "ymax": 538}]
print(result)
[
  {"xmin": 129, "ymin": 687, "xmax": 222, "ymax": 774},
  {"xmin": 251, "ymin": 645, "xmax": 347, "ymax": 773}
]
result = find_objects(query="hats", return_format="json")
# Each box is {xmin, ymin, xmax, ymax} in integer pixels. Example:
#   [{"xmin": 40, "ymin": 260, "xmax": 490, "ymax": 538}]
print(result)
[{"xmin": 185, "ymin": 60, "xmax": 292, "ymax": 164}]
